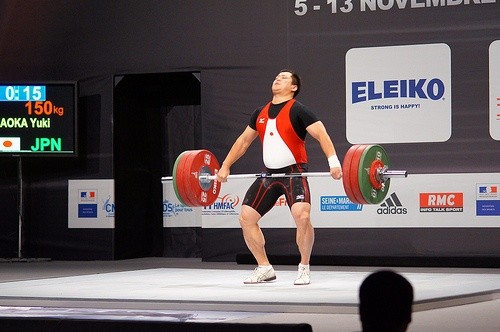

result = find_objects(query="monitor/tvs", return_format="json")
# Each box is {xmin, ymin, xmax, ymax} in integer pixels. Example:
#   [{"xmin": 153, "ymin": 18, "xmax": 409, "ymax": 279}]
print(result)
[{"xmin": 1, "ymin": 81, "xmax": 79, "ymax": 158}]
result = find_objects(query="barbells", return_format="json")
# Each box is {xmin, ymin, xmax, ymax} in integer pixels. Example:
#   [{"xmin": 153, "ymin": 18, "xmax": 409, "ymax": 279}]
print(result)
[{"xmin": 161, "ymin": 145, "xmax": 407, "ymax": 207}]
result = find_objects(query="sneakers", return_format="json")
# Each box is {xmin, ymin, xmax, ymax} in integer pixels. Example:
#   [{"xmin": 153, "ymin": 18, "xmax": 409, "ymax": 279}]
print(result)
[
  {"xmin": 294, "ymin": 263, "xmax": 311, "ymax": 285},
  {"xmin": 244, "ymin": 265, "xmax": 276, "ymax": 284}
]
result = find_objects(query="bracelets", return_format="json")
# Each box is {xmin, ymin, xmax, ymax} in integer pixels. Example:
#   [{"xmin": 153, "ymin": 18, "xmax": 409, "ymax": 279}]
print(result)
[{"xmin": 328, "ymin": 154, "xmax": 341, "ymax": 168}]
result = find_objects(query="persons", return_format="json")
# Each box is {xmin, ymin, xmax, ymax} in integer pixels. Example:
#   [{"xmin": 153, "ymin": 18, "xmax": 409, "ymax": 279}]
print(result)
[
  {"xmin": 359, "ymin": 270, "xmax": 414, "ymax": 332},
  {"xmin": 216, "ymin": 69, "xmax": 342, "ymax": 285}
]
